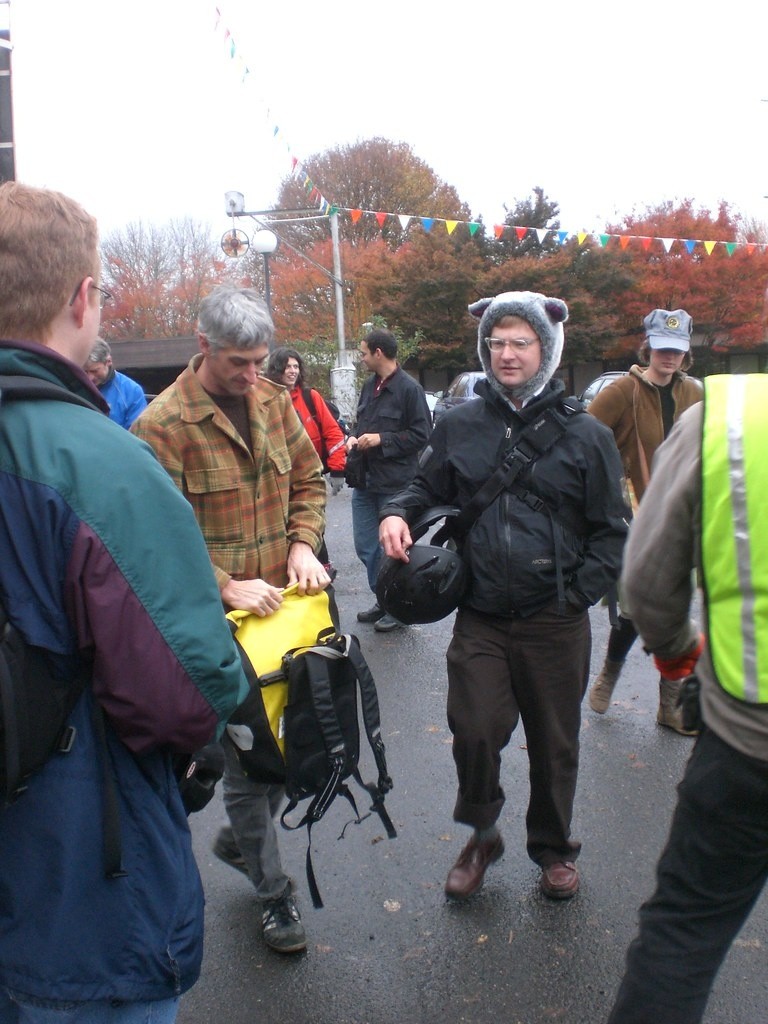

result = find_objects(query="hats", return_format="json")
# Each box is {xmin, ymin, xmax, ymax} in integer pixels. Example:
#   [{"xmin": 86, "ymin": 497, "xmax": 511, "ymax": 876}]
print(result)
[
  {"xmin": 643, "ymin": 308, "xmax": 694, "ymax": 352},
  {"xmin": 468, "ymin": 291, "xmax": 569, "ymax": 403}
]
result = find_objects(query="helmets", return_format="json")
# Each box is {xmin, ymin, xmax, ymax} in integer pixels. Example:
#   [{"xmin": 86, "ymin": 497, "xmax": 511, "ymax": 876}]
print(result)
[{"xmin": 376, "ymin": 505, "xmax": 471, "ymax": 625}]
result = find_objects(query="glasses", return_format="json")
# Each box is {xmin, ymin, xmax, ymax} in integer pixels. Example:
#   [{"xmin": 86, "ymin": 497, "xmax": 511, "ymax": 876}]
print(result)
[
  {"xmin": 70, "ymin": 274, "xmax": 111, "ymax": 310},
  {"xmin": 485, "ymin": 337, "xmax": 541, "ymax": 353}
]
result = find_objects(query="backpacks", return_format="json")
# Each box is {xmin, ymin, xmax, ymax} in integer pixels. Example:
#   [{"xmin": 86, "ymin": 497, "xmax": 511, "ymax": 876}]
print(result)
[
  {"xmin": 224, "ymin": 567, "xmax": 398, "ymax": 909},
  {"xmin": 0, "ymin": 375, "xmax": 126, "ymax": 884}
]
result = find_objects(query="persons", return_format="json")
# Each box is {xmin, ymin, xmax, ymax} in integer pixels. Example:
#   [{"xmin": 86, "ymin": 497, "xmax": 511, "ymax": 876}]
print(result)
[
  {"xmin": 72, "ymin": 332, "xmax": 149, "ymax": 433},
  {"xmin": 0, "ymin": 185, "xmax": 251, "ymax": 1024},
  {"xmin": 378, "ymin": 292, "xmax": 632, "ymax": 901},
  {"xmin": 127, "ymin": 285, "xmax": 333, "ymax": 959},
  {"xmin": 346, "ymin": 326, "xmax": 435, "ymax": 632},
  {"xmin": 266, "ymin": 348, "xmax": 347, "ymax": 496},
  {"xmin": 584, "ymin": 308, "xmax": 707, "ymax": 737},
  {"xmin": 605, "ymin": 369, "xmax": 768, "ymax": 1024}
]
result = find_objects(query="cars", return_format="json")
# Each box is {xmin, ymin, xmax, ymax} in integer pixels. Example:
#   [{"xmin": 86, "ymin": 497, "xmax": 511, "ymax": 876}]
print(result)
[{"xmin": 432, "ymin": 371, "xmax": 488, "ymax": 429}]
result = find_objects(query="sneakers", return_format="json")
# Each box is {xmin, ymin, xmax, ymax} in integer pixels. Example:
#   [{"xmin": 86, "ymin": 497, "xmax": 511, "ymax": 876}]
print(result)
[
  {"xmin": 261, "ymin": 879, "xmax": 306, "ymax": 953},
  {"xmin": 213, "ymin": 836, "xmax": 253, "ymax": 881}
]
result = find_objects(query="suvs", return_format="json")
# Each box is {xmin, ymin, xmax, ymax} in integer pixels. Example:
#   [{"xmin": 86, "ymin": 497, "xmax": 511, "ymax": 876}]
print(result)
[{"xmin": 569, "ymin": 371, "xmax": 629, "ymax": 410}]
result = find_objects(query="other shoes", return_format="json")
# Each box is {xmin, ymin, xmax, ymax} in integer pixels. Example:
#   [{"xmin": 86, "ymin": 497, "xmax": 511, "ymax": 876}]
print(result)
[
  {"xmin": 322, "ymin": 563, "xmax": 337, "ymax": 583},
  {"xmin": 357, "ymin": 604, "xmax": 384, "ymax": 621},
  {"xmin": 374, "ymin": 614, "xmax": 408, "ymax": 632}
]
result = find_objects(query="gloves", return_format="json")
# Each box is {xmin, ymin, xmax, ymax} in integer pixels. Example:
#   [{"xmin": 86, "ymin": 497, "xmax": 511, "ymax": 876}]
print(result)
[
  {"xmin": 330, "ymin": 476, "xmax": 344, "ymax": 496},
  {"xmin": 654, "ymin": 630, "xmax": 705, "ymax": 682}
]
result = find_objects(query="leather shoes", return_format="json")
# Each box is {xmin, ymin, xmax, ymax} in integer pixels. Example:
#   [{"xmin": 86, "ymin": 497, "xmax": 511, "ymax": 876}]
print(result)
[
  {"xmin": 444, "ymin": 832, "xmax": 505, "ymax": 900},
  {"xmin": 540, "ymin": 860, "xmax": 580, "ymax": 900}
]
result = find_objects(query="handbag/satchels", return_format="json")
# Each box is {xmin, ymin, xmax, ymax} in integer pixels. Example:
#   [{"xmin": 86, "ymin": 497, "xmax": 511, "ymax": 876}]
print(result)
[{"xmin": 302, "ymin": 386, "xmax": 340, "ymax": 475}]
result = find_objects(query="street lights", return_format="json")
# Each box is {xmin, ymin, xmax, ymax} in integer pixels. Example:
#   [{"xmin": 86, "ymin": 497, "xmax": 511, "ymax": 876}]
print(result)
[{"xmin": 253, "ymin": 230, "xmax": 277, "ymax": 315}]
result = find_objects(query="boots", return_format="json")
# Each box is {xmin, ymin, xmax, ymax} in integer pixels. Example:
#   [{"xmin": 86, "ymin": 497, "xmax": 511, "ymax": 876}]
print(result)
[
  {"xmin": 589, "ymin": 658, "xmax": 625, "ymax": 714},
  {"xmin": 656, "ymin": 678, "xmax": 698, "ymax": 736}
]
